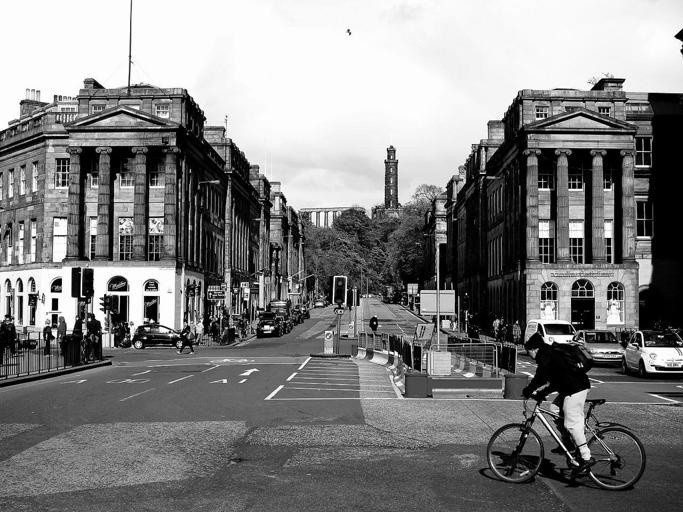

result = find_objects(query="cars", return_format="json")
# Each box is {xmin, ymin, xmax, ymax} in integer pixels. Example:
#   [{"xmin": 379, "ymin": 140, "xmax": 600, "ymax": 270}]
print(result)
[
  {"xmin": 524, "ymin": 319, "xmax": 683, "ymax": 378},
  {"xmin": 132, "ymin": 324, "xmax": 183, "ymax": 349},
  {"xmin": 314, "ymin": 299, "xmax": 325, "ymax": 308},
  {"xmin": 257, "ymin": 301, "xmax": 310, "ymax": 339}
]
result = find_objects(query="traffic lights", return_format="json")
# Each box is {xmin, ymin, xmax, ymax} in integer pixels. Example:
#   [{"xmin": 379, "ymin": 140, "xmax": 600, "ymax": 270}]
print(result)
[
  {"xmin": 335, "ymin": 277, "xmax": 345, "ymax": 304},
  {"xmin": 105, "ymin": 297, "xmax": 111, "ymax": 311}
]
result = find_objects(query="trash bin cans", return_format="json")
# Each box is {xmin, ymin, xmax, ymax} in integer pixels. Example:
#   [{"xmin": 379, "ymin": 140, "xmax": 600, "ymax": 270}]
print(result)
[
  {"xmin": 324, "ymin": 330, "xmax": 334, "ymax": 353},
  {"xmin": 61, "ymin": 334, "xmax": 80, "ymax": 366},
  {"xmin": 468, "ymin": 324, "xmax": 480, "ymax": 343}
]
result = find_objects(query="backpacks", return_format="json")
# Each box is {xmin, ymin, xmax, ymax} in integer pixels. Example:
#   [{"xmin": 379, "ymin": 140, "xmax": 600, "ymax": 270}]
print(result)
[{"xmin": 553, "ymin": 341, "xmax": 596, "ymax": 374}]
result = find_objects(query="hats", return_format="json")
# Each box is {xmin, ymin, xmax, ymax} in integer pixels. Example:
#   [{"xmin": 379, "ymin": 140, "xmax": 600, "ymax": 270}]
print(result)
[{"xmin": 524, "ymin": 332, "xmax": 544, "ymax": 351}]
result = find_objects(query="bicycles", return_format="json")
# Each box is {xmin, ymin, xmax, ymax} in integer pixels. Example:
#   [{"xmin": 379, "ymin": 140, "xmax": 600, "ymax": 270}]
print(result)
[{"xmin": 487, "ymin": 393, "xmax": 647, "ymax": 490}]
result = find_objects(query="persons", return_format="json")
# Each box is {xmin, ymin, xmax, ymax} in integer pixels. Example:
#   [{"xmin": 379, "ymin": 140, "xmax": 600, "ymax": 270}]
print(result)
[
  {"xmin": 450, "ymin": 306, "xmax": 521, "ymax": 346},
  {"xmin": 0, "ymin": 307, "xmax": 251, "ymax": 365},
  {"xmin": 520, "ymin": 332, "xmax": 596, "ymax": 480},
  {"xmin": 620, "ymin": 327, "xmax": 629, "ymax": 348}
]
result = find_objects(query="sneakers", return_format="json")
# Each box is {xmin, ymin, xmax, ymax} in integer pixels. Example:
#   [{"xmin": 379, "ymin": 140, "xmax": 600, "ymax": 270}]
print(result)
[
  {"xmin": 572, "ymin": 455, "xmax": 596, "ymax": 476},
  {"xmin": 550, "ymin": 439, "xmax": 575, "ymax": 453}
]
